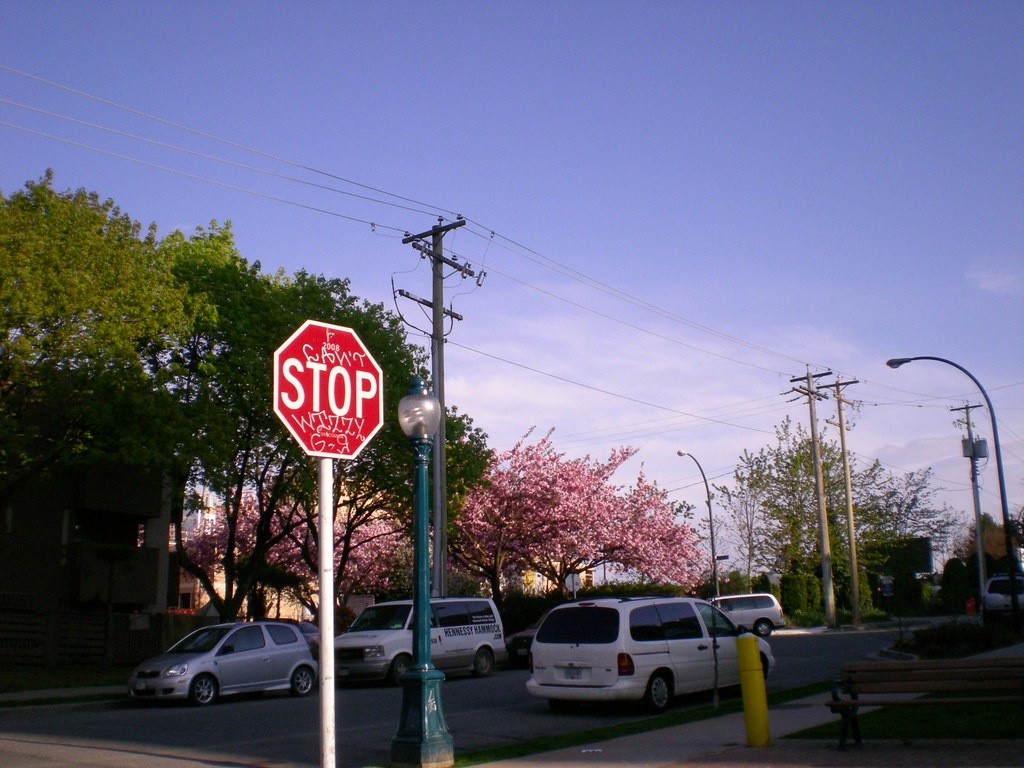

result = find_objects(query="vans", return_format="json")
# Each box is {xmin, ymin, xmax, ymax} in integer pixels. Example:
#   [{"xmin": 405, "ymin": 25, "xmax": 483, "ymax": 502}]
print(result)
[
  {"xmin": 675, "ymin": 593, "xmax": 786, "ymax": 637},
  {"xmin": 334, "ymin": 598, "xmax": 507, "ymax": 688},
  {"xmin": 526, "ymin": 596, "xmax": 778, "ymax": 710}
]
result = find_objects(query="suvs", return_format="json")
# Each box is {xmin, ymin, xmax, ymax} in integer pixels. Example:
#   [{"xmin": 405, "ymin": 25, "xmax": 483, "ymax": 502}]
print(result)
[{"xmin": 983, "ymin": 575, "xmax": 1023, "ymax": 613}]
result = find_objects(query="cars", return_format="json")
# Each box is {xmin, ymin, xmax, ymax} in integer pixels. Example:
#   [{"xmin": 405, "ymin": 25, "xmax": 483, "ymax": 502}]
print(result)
[
  {"xmin": 287, "ymin": 621, "xmax": 319, "ymax": 660},
  {"xmin": 505, "ymin": 612, "xmax": 551, "ymax": 664},
  {"xmin": 125, "ymin": 622, "xmax": 320, "ymax": 703}
]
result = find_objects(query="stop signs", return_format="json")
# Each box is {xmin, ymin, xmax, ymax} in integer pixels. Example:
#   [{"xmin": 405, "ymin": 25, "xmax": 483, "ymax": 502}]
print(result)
[{"xmin": 274, "ymin": 319, "xmax": 385, "ymax": 460}]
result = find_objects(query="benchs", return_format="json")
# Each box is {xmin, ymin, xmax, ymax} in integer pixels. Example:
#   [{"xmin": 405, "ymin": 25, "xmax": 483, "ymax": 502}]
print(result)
[{"xmin": 826, "ymin": 659, "xmax": 1024, "ymax": 750}]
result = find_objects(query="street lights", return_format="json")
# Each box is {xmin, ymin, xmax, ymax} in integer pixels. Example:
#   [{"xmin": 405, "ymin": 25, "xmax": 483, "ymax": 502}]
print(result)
[
  {"xmin": 391, "ymin": 372, "xmax": 454, "ymax": 767},
  {"xmin": 886, "ymin": 355, "xmax": 1021, "ymax": 619},
  {"xmin": 676, "ymin": 450, "xmax": 720, "ymax": 608}
]
